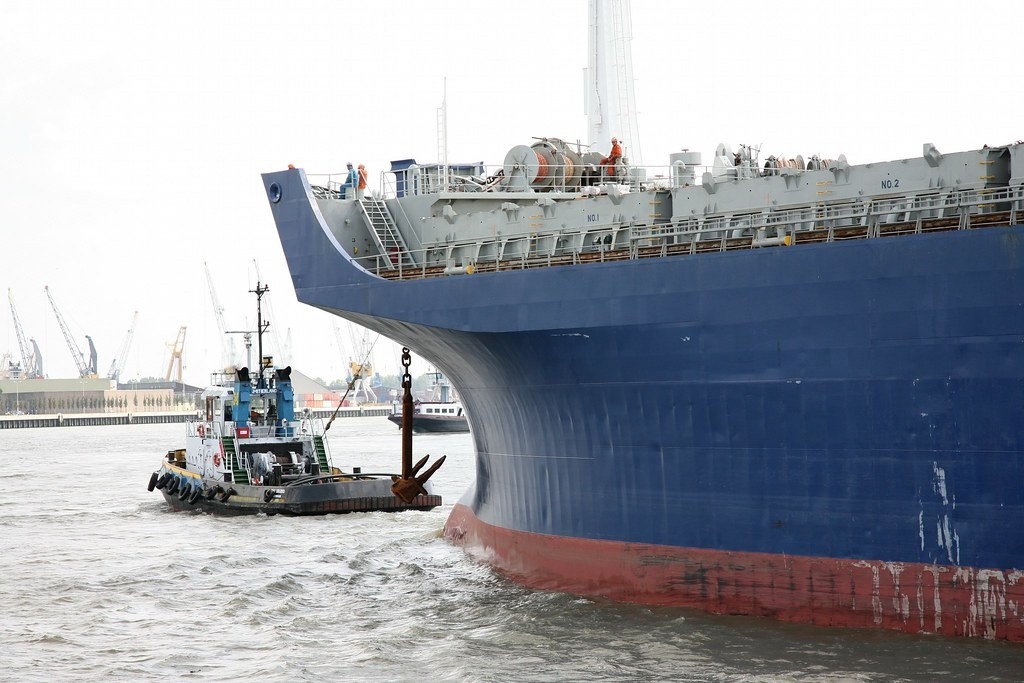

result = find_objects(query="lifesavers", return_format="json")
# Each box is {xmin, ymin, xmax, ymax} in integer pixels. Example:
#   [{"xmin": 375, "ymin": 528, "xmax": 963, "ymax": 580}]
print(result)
[
  {"xmin": 219, "ymin": 490, "xmax": 232, "ymax": 503},
  {"xmin": 148, "ymin": 472, "xmax": 158, "ymax": 492},
  {"xmin": 213, "ymin": 453, "xmax": 220, "ymax": 467},
  {"xmin": 205, "ymin": 485, "xmax": 216, "ymax": 499},
  {"xmin": 188, "ymin": 486, "xmax": 202, "ymax": 504},
  {"xmin": 197, "ymin": 424, "xmax": 205, "ymax": 437},
  {"xmin": 166, "ymin": 476, "xmax": 180, "ymax": 495},
  {"xmin": 178, "ymin": 483, "xmax": 192, "ymax": 500},
  {"xmin": 156, "ymin": 472, "xmax": 170, "ymax": 489},
  {"xmin": 263, "ymin": 488, "xmax": 275, "ymax": 502}
]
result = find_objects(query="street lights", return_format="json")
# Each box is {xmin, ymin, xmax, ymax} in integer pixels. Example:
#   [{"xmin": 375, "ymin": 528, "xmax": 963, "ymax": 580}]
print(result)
[
  {"xmin": 13, "ymin": 381, "xmax": 23, "ymax": 411},
  {"xmin": 79, "ymin": 381, "xmax": 87, "ymax": 413}
]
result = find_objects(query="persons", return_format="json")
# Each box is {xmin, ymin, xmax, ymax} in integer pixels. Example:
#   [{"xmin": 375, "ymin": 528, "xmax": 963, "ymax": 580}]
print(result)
[
  {"xmin": 288, "ymin": 164, "xmax": 294, "ymax": 169},
  {"xmin": 252, "ymin": 472, "xmax": 264, "ymax": 486},
  {"xmin": 338, "ymin": 162, "xmax": 358, "ymax": 199},
  {"xmin": 357, "ymin": 164, "xmax": 367, "ymax": 189},
  {"xmin": 600, "ymin": 138, "xmax": 622, "ymax": 182}
]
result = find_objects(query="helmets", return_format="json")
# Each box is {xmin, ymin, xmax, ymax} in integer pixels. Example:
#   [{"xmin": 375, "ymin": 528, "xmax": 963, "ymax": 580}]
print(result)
[
  {"xmin": 346, "ymin": 161, "xmax": 353, "ymax": 165},
  {"xmin": 358, "ymin": 163, "xmax": 364, "ymax": 168},
  {"xmin": 287, "ymin": 163, "xmax": 295, "ymax": 168},
  {"xmin": 611, "ymin": 137, "xmax": 618, "ymax": 142}
]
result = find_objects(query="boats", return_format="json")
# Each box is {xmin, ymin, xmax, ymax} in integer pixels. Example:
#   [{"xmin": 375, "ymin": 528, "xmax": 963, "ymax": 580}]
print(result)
[
  {"xmin": 149, "ymin": 283, "xmax": 433, "ymax": 517},
  {"xmin": 264, "ymin": 0, "xmax": 1024, "ymax": 645},
  {"xmin": 388, "ymin": 366, "xmax": 469, "ymax": 433}
]
